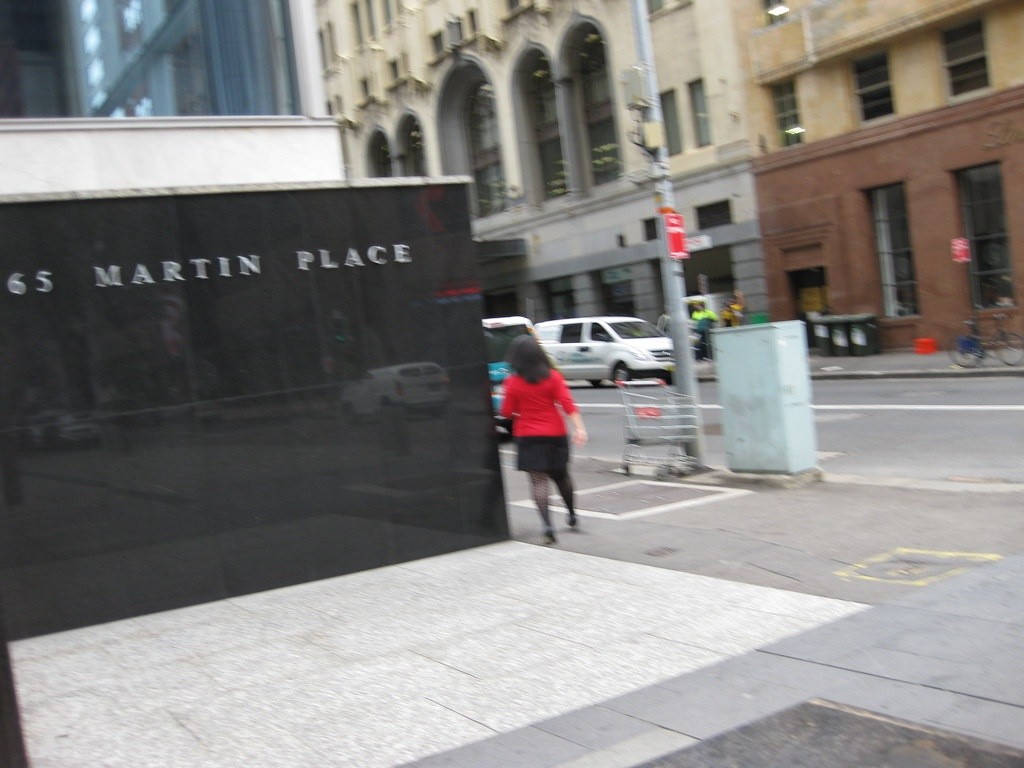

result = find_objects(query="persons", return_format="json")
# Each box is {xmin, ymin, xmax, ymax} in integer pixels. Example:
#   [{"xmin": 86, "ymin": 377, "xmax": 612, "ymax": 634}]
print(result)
[
  {"xmin": 692, "ymin": 302, "xmax": 719, "ymax": 361},
  {"xmin": 722, "ymin": 296, "xmax": 743, "ymax": 327},
  {"xmin": 500, "ymin": 334, "xmax": 588, "ymax": 545}
]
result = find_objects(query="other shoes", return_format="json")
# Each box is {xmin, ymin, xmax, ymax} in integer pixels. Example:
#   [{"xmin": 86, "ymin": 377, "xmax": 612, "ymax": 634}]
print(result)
[
  {"xmin": 544, "ymin": 530, "xmax": 555, "ymax": 543},
  {"xmin": 569, "ymin": 515, "xmax": 576, "ymax": 526}
]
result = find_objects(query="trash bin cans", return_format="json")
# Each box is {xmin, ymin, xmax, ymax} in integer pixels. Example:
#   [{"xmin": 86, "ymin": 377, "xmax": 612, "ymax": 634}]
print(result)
[{"xmin": 810, "ymin": 312, "xmax": 880, "ymax": 358}]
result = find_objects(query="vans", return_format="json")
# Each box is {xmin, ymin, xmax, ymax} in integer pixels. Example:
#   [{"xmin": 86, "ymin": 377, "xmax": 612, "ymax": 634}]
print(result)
[{"xmin": 533, "ymin": 316, "xmax": 673, "ymax": 388}]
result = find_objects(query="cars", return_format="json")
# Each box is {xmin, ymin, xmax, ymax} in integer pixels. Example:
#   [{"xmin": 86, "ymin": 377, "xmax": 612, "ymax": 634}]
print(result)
[
  {"xmin": 369, "ymin": 361, "xmax": 450, "ymax": 420},
  {"xmin": 14, "ymin": 409, "xmax": 65, "ymax": 452},
  {"xmin": 653, "ymin": 294, "xmax": 730, "ymax": 349},
  {"xmin": 483, "ymin": 316, "xmax": 542, "ymax": 434},
  {"xmin": 57, "ymin": 412, "xmax": 104, "ymax": 450}
]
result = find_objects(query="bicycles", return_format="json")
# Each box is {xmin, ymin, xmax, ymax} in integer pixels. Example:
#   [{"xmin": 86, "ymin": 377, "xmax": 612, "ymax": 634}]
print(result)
[{"xmin": 947, "ymin": 313, "xmax": 1023, "ymax": 369}]
